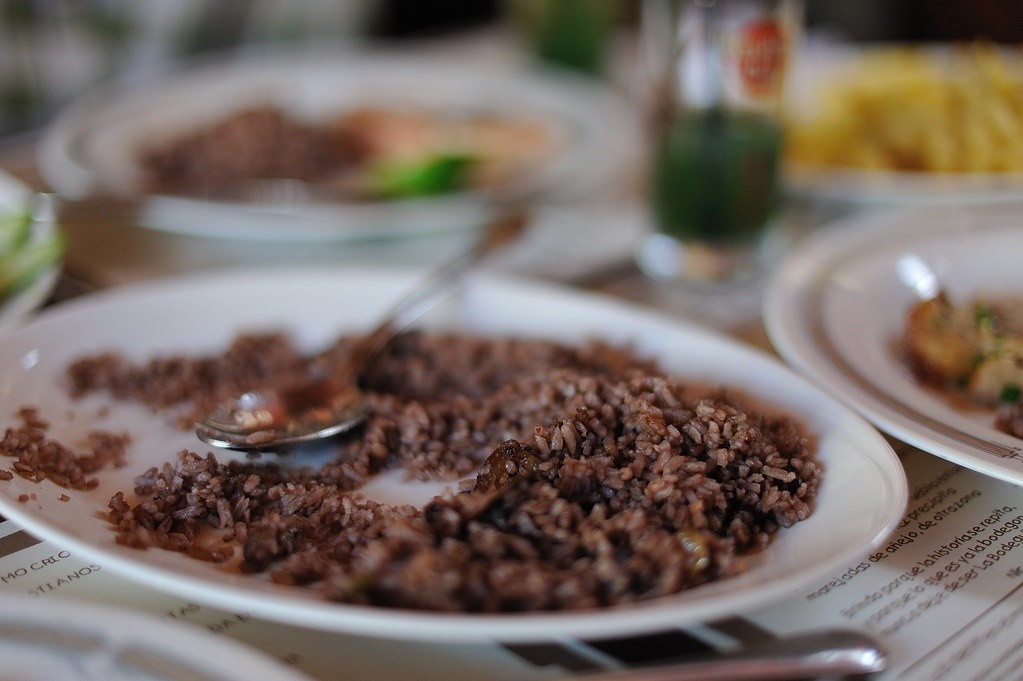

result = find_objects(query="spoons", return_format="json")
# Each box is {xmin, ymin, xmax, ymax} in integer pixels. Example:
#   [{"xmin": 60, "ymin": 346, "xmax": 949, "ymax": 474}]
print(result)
[{"xmin": 193, "ymin": 211, "xmax": 528, "ymax": 452}]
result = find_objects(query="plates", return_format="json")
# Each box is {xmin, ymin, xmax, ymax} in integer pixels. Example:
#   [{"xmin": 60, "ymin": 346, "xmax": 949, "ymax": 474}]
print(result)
[
  {"xmin": 0, "ymin": 595, "xmax": 309, "ymax": 681},
  {"xmin": 0, "ymin": 264, "xmax": 911, "ymax": 644},
  {"xmin": 763, "ymin": 198, "xmax": 1023, "ymax": 485},
  {"xmin": 32, "ymin": 46, "xmax": 650, "ymax": 243},
  {"xmin": 777, "ymin": 31, "xmax": 1023, "ymax": 204},
  {"xmin": 0, "ymin": 178, "xmax": 58, "ymax": 331}
]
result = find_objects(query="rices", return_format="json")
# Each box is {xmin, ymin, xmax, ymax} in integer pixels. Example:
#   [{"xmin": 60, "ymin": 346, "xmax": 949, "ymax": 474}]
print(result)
[{"xmin": 0, "ymin": 330, "xmax": 821, "ymax": 612}]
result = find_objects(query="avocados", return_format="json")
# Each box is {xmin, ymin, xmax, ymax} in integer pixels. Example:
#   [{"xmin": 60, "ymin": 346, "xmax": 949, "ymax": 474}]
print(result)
[{"xmin": 363, "ymin": 147, "xmax": 475, "ymax": 198}]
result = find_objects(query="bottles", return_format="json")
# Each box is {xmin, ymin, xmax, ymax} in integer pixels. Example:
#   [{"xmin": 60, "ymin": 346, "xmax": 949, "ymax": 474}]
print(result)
[{"xmin": 656, "ymin": 0, "xmax": 798, "ymax": 243}]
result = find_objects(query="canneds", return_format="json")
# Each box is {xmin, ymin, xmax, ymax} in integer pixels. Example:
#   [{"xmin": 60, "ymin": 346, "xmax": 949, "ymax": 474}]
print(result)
[{"xmin": 628, "ymin": 0, "xmax": 804, "ymax": 282}]
